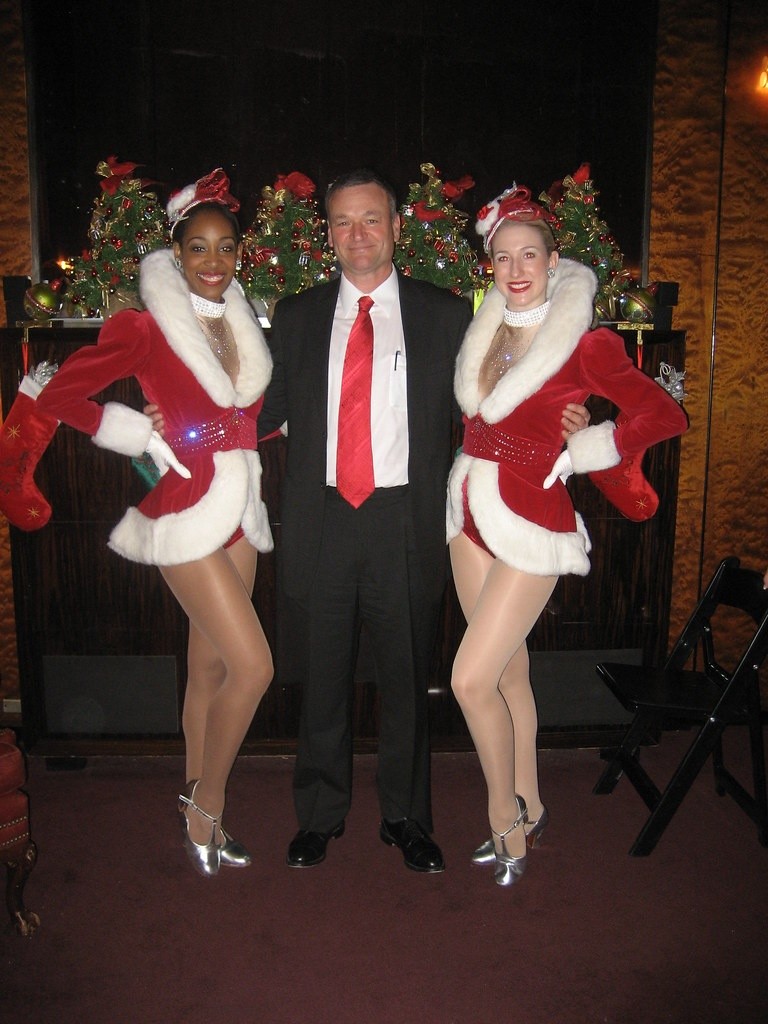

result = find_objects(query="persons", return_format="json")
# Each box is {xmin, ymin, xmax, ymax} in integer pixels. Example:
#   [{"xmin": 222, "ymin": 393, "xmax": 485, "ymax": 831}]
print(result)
[
  {"xmin": 446, "ymin": 191, "xmax": 688, "ymax": 887},
  {"xmin": 143, "ymin": 163, "xmax": 591, "ymax": 872},
  {"xmin": 34, "ymin": 172, "xmax": 275, "ymax": 878}
]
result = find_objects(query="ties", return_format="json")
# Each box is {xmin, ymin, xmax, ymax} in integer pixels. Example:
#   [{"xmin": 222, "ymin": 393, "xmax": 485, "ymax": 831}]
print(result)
[{"xmin": 336, "ymin": 297, "xmax": 375, "ymax": 510}]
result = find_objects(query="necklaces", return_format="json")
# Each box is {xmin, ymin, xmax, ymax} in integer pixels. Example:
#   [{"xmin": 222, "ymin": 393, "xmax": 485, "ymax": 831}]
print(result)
[
  {"xmin": 189, "ymin": 293, "xmax": 226, "ymax": 319},
  {"xmin": 503, "ymin": 300, "xmax": 548, "ymax": 327}
]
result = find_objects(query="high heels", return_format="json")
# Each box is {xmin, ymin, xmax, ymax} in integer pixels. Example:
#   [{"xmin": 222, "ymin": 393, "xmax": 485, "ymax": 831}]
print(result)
[
  {"xmin": 178, "ymin": 780, "xmax": 222, "ymax": 879},
  {"xmin": 219, "ymin": 828, "xmax": 253, "ymax": 869},
  {"xmin": 470, "ymin": 803, "xmax": 550, "ymax": 867},
  {"xmin": 488, "ymin": 794, "xmax": 528, "ymax": 887}
]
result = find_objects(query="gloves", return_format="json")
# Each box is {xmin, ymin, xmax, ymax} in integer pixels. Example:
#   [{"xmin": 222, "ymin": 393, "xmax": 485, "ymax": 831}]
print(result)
[
  {"xmin": 145, "ymin": 431, "xmax": 192, "ymax": 480},
  {"xmin": 543, "ymin": 451, "xmax": 574, "ymax": 490}
]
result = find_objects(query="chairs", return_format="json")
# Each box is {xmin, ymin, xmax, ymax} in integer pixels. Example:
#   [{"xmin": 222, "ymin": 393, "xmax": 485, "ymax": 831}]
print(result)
[{"xmin": 594, "ymin": 556, "xmax": 768, "ymax": 857}]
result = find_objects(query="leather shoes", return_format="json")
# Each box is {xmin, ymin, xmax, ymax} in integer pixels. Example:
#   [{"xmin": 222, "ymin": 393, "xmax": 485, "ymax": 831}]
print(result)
[
  {"xmin": 286, "ymin": 816, "xmax": 346, "ymax": 867},
  {"xmin": 379, "ymin": 816, "xmax": 446, "ymax": 873}
]
